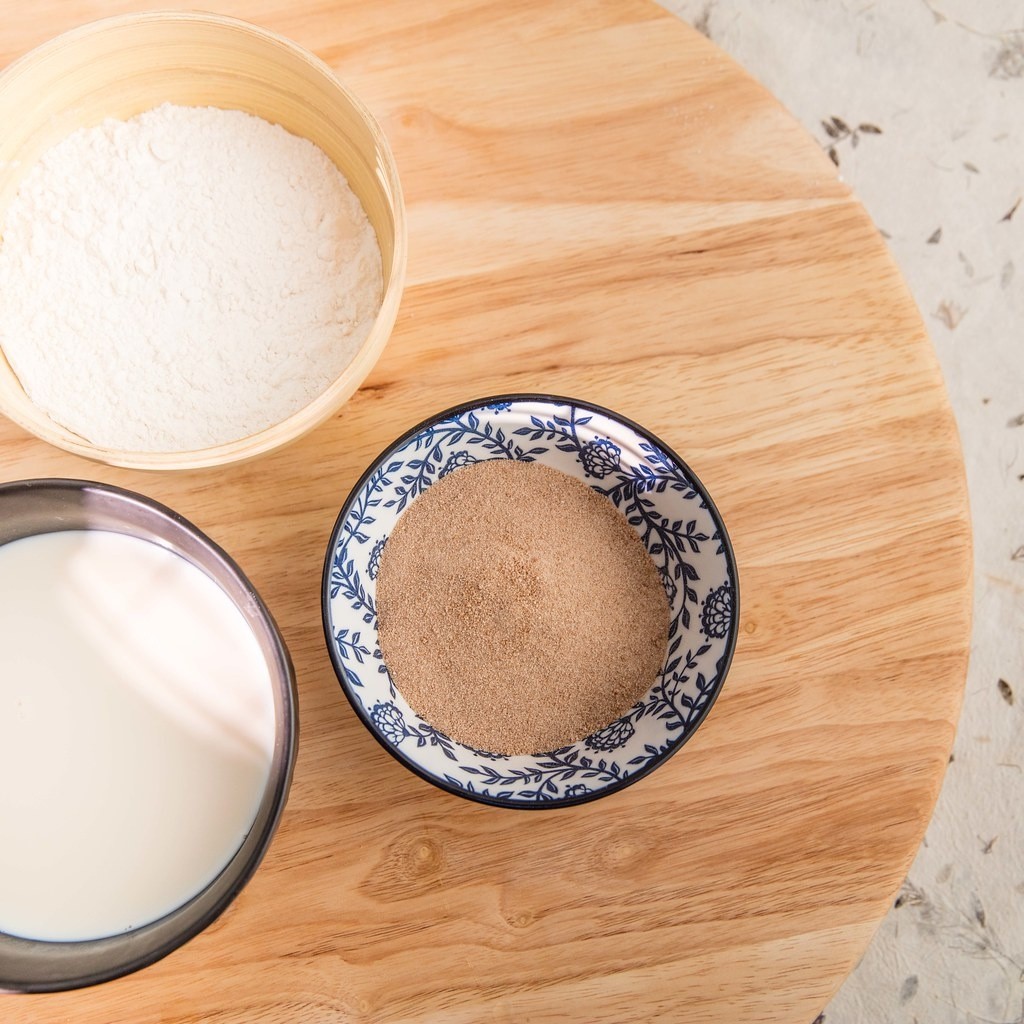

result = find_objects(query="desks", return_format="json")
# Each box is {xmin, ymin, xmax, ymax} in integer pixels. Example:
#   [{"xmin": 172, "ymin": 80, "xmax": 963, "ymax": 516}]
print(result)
[{"xmin": 0, "ymin": 0, "xmax": 979, "ymax": 1023}]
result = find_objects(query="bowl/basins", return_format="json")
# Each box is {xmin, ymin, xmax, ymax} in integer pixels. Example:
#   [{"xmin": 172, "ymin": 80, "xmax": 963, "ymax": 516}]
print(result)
[
  {"xmin": 320, "ymin": 393, "xmax": 741, "ymax": 810},
  {"xmin": 0, "ymin": 478, "xmax": 300, "ymax": 993},
  {"xmin": 0, "ymin": 10, "xmax": 407, "ymax": 471}
]
